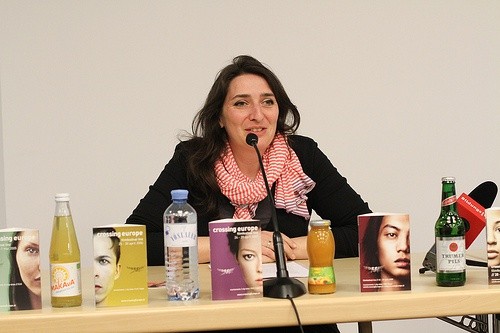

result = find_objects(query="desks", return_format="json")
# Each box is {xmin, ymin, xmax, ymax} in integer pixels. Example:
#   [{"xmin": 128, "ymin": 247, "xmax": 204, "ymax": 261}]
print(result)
[{"xmin": 0, "ymin": 249, "xmax": 500, "ymax": 333}]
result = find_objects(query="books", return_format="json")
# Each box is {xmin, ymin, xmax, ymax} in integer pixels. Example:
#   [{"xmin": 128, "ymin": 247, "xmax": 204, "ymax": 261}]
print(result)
[
  {"xmin": 0, "ymin": 227, "xmax": 42, "ymax": 312},
  {"xmin": 357, "ymin": 211, "xmax": 412, "ymax": 292},
  {"xmin": 208, "ymin": 219, "xmax": 264, "ymax": 300},
  {"xmin": 92, "ymin": 225, "xmax": 149, "ymax": 308},
  {"xmin": 484, "ymin": 207, "xmax": 500, "ymax": 285}
]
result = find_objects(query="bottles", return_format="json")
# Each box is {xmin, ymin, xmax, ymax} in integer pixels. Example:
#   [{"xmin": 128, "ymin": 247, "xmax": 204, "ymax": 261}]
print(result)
[
  {"xmin": 435, "ymin": 176, "xmax": 467, "ymax": 287},
  {"xmin": 48, "ymin": 192, "xmax": 83, "ymax": 307},
  {"xmin": 307, "ymin": 220, "xmax": 337, "ymax": 294},
  {"xmin": 163, "ymin": 189, "xmax": 200, "ymax": 302}
]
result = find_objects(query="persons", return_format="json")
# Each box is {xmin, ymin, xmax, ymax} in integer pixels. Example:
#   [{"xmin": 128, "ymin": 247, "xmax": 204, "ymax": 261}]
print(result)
[
  {"xmin": 362, "ymin": 215, "xmax": 411, "ymax": 292},
  {"xmin": 125, "ymin": 55, "xmax": 373, "ymax": 333},
  {"xmin": 92, "ymin": 228, "xmax": 123, "ymax": 304},
  {"xmin": 227, "ymin": 222, "xmax": 263, "ymax": 298},
  {"xmin": 8, "ymin": 232, "xmax": 42, "ymax": 311},
  {"xmin": 486, "ymin": 210, "xmax": 500, "ymax": 266}
]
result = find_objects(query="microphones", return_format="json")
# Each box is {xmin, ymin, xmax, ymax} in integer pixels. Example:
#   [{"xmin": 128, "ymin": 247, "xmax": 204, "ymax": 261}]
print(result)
[
  {"xmin": 455, "ymin": 182, "xmax": 497, "ymax": 249},
  {"xmin": 246, "ymin": 133, "xmax": 307, "ymax": 298}
]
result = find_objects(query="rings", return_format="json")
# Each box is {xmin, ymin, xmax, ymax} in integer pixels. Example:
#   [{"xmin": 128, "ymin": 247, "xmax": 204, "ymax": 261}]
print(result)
[{"xmin": 266, "ymin": 241, "xmax": 271, "ymax": 248}]
